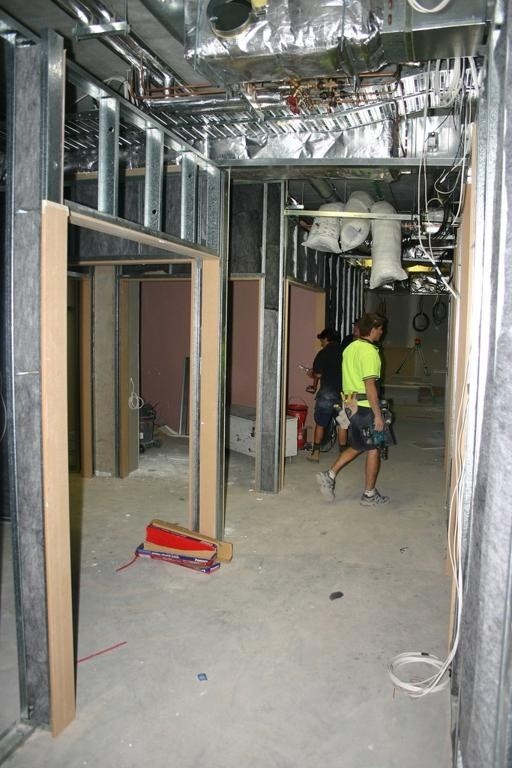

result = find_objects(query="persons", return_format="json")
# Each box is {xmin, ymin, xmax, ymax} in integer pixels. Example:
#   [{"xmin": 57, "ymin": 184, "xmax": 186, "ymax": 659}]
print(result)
[
  {"xmin": 306, "ymin": 328, "xmax": 346, "ymax": 463},
  {"xmin": 339, "ymin": 317, "xmax": 359, "ymax": 348},
  {"xmin": 314, "ymin": 312, "xmax": 391, "ymax": 508}
]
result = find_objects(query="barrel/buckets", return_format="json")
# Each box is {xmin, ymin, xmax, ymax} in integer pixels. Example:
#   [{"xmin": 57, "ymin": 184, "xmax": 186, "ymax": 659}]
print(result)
[
  {"xmin": 140, "ymin": 415, "xmax": 155, "ymax": 442},
  {"xmin": 287, "ymin": 395, "xmax": 309, "ymax": 431}
]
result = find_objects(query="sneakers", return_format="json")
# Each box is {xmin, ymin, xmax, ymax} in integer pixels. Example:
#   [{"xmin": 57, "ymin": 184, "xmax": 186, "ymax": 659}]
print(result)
[
  {"xmin": 360, "ymin": 488, "xmax": 390, "ymax": 506},
  {"xmin": 306, "ymin": 450, "xmax": 319, "ymax": 464},
  {"xmin": 316, "ymin": 470, "xmax": 335, "ymax": 502}
]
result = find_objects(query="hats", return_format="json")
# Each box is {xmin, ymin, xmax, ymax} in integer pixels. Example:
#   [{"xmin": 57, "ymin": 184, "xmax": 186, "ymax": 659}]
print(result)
[
  {"xmin": 352, "ymin": 318, "xmax": 361, "ymax": 324},
  {"xmin": 317, "ymin": 327, "xmax": 340, "ymax": 341}
]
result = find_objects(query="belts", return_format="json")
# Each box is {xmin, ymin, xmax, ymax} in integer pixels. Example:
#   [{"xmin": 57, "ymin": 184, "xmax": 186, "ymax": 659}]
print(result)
[{"xmin": 345, "ymin": 394, "xmax": 367, "ymax": 401}]
